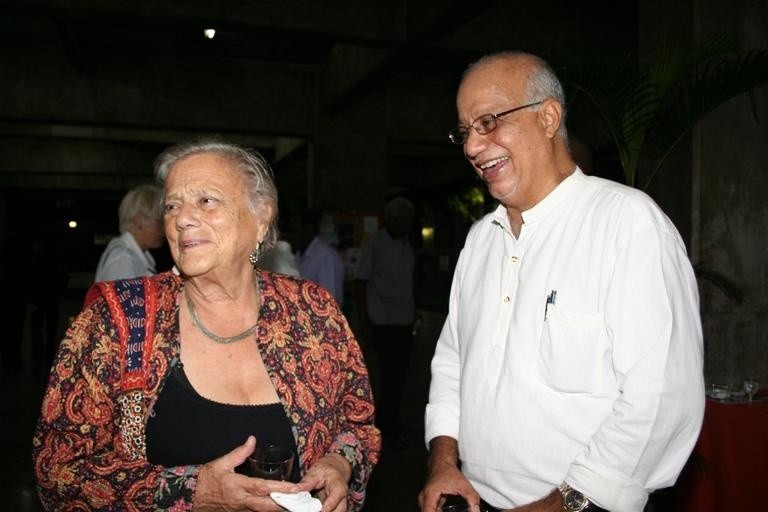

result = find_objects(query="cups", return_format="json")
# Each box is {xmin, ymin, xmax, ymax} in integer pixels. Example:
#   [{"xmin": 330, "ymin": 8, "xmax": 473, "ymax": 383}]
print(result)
[{"xmin": 245, "ymin": 445, "xmax": 296, "ymax": 484}]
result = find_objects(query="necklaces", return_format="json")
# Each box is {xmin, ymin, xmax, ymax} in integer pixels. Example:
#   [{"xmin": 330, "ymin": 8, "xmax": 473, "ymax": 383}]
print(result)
[{"xmin": 176, "ymin": 280, "xmax": 261, "ymax": 345}]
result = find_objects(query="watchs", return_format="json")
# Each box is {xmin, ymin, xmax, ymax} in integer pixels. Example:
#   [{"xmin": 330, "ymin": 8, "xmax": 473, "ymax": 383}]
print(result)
[{"xmin": 555, "ymin": 481, "xmax": 590, "ymax": 512}]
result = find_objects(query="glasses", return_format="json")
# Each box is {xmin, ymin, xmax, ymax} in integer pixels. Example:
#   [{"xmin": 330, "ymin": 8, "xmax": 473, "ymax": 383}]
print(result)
[{"xmin": 448, "ymin": 101, "xmax": 544, "ymax": 146}]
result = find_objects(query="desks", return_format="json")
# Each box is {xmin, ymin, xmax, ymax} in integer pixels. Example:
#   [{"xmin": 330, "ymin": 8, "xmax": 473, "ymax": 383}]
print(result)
[{"xmin": 684, "ymin": 383, "xmax": 768, "ymax": 512}]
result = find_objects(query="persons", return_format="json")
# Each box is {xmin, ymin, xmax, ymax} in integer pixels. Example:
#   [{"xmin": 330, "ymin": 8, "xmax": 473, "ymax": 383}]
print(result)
[
  {"xmin": 293, "ymin": 210, "xmax": 344, "ymax": 302},
  {"xmin": 91, "ymin": 186, "xmax": 165, "ymax": 283},
  {"xmin": 417, "ymin": 48, "xmax": 707, "ymax": 512},
  {"xmin": 30, "ymin": 140, "xmax": 381, "ymax": 512},
  {"xmin": 356, "ymin": 197, "xmax": 417, "ymax": 374}
]
